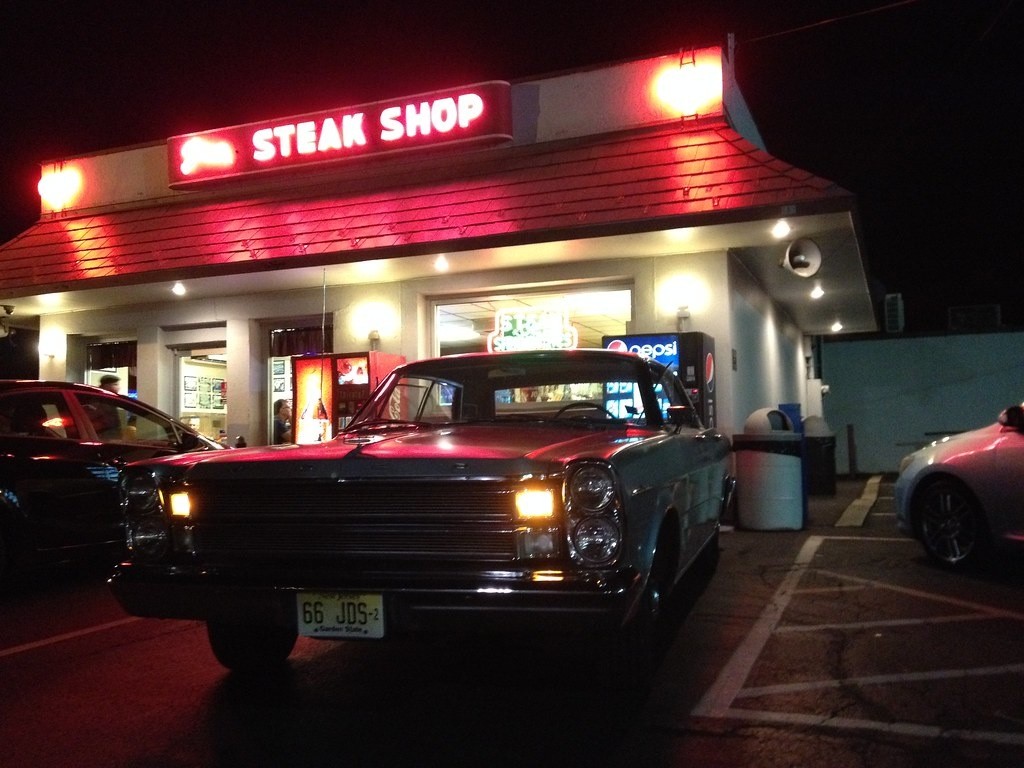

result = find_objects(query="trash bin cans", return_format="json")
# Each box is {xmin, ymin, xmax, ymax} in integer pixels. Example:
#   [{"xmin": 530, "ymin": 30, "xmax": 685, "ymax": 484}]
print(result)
[
  {"xmin": 729, "ymin": 406, "xmax": 806, "ymax": 529},
  {"xmin": 800, "ymin": 415, "xmax": 840, "ymax": 500}
]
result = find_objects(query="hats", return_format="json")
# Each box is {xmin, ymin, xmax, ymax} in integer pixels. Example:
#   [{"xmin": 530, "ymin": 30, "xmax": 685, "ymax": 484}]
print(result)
[{"xmin": 100, "ymin": 375, "xmax": 119, "ymax": 384}]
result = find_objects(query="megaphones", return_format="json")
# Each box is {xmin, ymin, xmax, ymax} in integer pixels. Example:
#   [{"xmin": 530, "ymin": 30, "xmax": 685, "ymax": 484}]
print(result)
[{"xmin": 777, "ymin": 236, "xmax": 825, "ymax": 279}]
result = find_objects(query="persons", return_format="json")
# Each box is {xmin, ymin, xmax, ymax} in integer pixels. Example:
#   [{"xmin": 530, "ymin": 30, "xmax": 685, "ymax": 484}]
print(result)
[
  {"xmin": 274, "ymin": 399, "xmax": 292, "ymax": 444},
  {"xmin": 95, "ymin": 375, "xmax": 121, "ymax": 441}
]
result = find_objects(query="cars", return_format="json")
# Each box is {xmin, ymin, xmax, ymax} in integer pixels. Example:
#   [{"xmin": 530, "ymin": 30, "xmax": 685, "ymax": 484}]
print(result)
[
  {"xmin": 107, "ymin": 349, "xmax": 740, "ymax": 691},
  {"xmin": 894, "ymin": 400, "xmax": 1024, "ymax": 572},
  {"xmin": 0, "ymin": 380, "xmax": 236, "ymax": 596}
]
